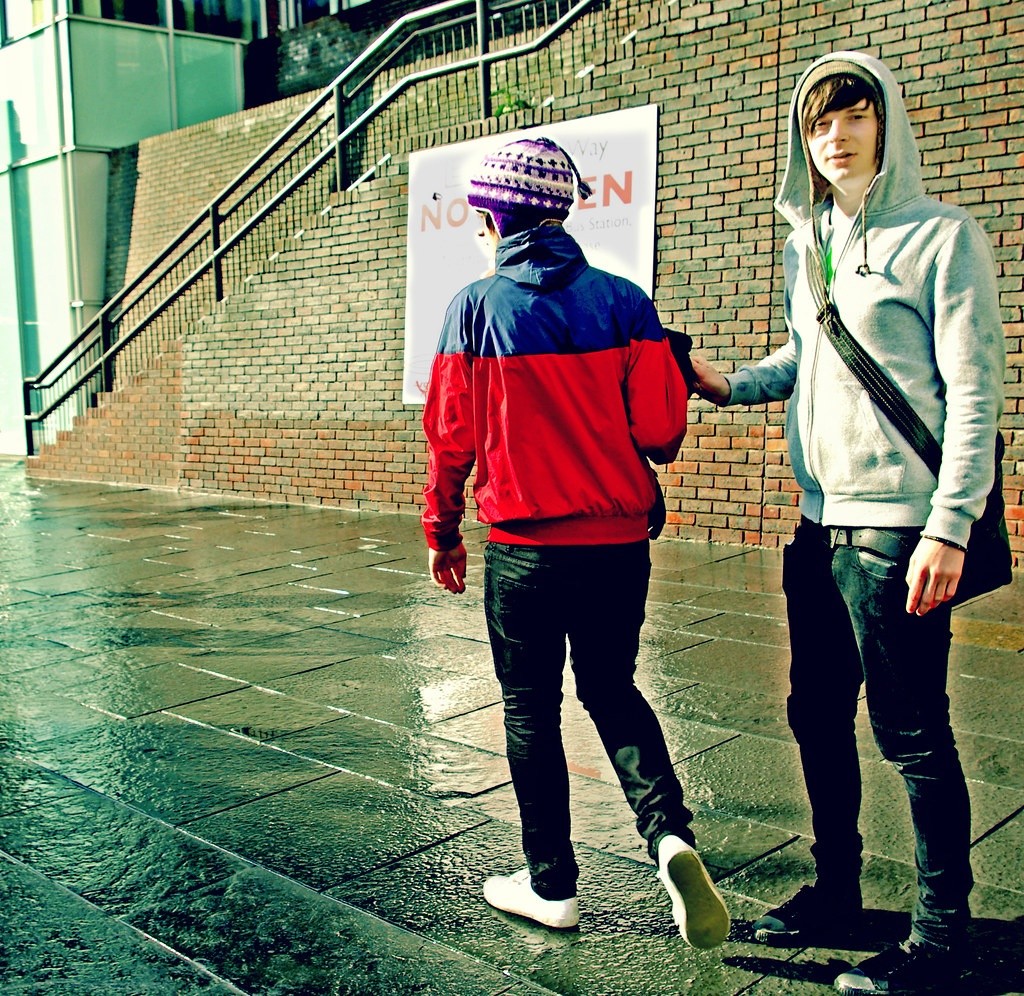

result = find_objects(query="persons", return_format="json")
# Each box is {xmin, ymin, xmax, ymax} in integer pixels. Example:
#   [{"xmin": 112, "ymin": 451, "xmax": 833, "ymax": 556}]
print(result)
[
  {"xmin": 690, "ymin": 47, "xmax": 1004, "ymax": 996},
  {"xmin": 419, "ymin": 136, "xmax": 732, "ymax": 948}
]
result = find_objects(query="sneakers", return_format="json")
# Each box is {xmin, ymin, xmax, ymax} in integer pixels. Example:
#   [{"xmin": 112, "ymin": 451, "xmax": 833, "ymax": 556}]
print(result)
[
  {"xmin": 833, "ymin": 943, "xmax": 976, "ymax": 996},
  {"xmin": 750, "ymin": 884, "xmax": 866, "ymax": 945}
]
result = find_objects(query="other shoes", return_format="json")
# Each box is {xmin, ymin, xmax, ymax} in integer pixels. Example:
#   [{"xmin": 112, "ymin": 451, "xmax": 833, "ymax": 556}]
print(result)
[
  {"xmin": 482, "ymin": 869, "xmax": 579, "ymax": 929},
  {"xmin": 654, "ymin": 836, "xmax": 731, "ymax": 951}
]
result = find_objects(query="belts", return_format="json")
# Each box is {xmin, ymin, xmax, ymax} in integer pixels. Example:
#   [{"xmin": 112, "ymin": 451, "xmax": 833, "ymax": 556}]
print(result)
[{"xmin": 801, "ymin": 516, "xmax": 909, "ymax": 560}]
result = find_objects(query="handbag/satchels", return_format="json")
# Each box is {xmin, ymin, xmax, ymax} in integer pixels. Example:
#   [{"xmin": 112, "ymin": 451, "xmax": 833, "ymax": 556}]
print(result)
[{"xmin": 950, "ymin": 429, "xmax": 1014, "ymax": 610}]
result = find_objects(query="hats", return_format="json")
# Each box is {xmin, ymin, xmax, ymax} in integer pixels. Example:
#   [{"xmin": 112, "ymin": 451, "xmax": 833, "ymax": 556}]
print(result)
[
  {"xmin": 466, "ymin": 137, "xmax": 593, "ymax": 239},
  {"xmin": 795, "ymin": 58, "xmax": 886, "ymax": 334}
]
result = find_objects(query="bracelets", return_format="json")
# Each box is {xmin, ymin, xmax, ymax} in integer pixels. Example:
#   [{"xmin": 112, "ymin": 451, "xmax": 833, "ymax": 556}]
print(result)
[{"xmin": 925, "ymin": 535, "xmax": 968, "ymax": 553}]
